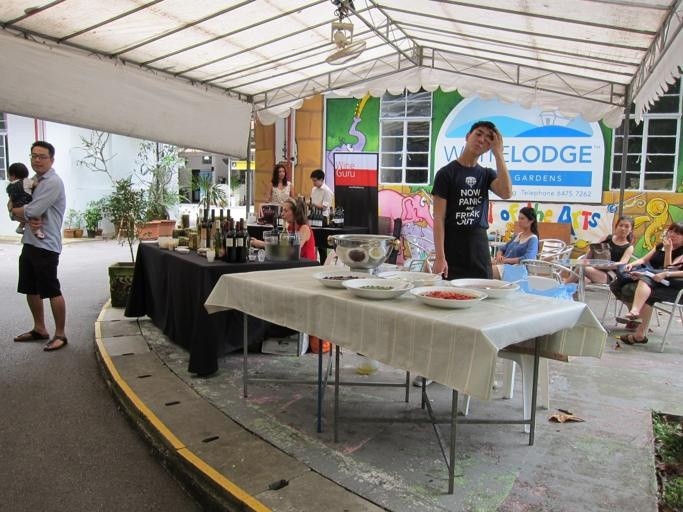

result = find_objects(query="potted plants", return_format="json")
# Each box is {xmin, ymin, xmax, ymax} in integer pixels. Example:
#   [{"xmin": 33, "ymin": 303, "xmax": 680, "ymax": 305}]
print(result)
[{"xmin": 63, "ymin": 154, "xmax": 191, "ymax": 308}]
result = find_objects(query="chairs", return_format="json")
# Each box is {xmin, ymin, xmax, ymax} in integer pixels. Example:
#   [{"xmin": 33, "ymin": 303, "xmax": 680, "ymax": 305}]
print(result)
[
  {"xmin": 489, "ymin": 239, "xmax": 683, "ymax": 352},
  {"xmin": 404, "ymin": 235, "xmax": 435, "ymax": 272}
]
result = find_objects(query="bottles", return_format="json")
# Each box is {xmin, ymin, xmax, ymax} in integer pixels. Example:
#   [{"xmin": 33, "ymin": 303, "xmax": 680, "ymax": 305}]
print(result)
[
  {"xmin": 236, "ymin": 222, "xmax": 241, "ymax": 232},
  {"xmin": 227, "ymin": 209, "xmax": 231, "ymax": 219},
  {"xmin": 199, "ymin": 209, "xmax": 209, "ymax": 248},
  {"xmin": 219, "ymin": 208, "xmax": 223, "ymax": 220},
  {"xmin": 329, "ymin": 206, "xmax": 345, "ymax": 228},
  {"xmin": 279, "ymin": 217, "xmax": 289, "ymax": 260},
  {"xmin": 308, "ymin": 207, "xmax": 326, "ymax": 227},
  {"xmin": 225, "ymin": 221, "xmax": 237, "ymax": 263},
  {"xmin": 288, "ymin": 221, "xmax": 300, "ymax": 262},
  {"xmin": 270, "ymin": 217, "xmax": 280, "ymax": 261},
  {"xmin": 228, "ymin": 218, "xmax": 236, "ymax": 236},
  {"xmin": 214, "ymin": 228, "xmax": 221, "ymax": 258},
  {"xmin": 236, "ymin": 217, "xmax": 249, "ymax": 262},
  {"xmin": 207, "ymin": 209, "xmax": 217, "ymax": 248}
]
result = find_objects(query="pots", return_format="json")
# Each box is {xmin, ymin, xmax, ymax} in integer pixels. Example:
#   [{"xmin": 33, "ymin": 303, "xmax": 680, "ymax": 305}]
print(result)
[{"xmin": 326, "ymin": 233, "xmax": 400, "ymax": 275}]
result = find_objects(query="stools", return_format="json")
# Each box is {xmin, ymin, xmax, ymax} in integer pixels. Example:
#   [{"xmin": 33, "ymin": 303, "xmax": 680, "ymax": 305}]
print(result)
[{"xmin": 461, "ymin": 277, "xmax": 573, "ymax": 434}]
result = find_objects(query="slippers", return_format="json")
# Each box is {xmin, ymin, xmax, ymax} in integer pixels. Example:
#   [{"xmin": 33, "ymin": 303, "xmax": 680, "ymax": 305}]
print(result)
[
  {"xmin": 44, "ymin": 335, "xmax": 67, "ymax": 351},
  {"xmin": 14, "ymin": 330, "xmax": 49, "ymax": 342}
]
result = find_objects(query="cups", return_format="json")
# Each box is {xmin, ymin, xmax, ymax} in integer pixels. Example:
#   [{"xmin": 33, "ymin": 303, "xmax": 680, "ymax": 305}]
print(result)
[{"xmin": 207, "ymin": 251, "xmax": 215, "ymax": 262}]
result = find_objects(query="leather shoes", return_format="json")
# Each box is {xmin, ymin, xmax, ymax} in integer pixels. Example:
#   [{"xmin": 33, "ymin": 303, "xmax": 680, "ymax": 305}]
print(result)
[
  {"xmin": 616, "ymin": 312, "xmax": 642, "ymax": 324},
  {"xmin": 620, "ymin": 334, "xmax": 648, "ymax": 345}
]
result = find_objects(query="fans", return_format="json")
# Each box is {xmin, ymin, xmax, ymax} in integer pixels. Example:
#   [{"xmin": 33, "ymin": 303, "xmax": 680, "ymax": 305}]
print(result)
[{"xmin": 324, "ymin": 0, "xmax": 366, "ymax": 67}]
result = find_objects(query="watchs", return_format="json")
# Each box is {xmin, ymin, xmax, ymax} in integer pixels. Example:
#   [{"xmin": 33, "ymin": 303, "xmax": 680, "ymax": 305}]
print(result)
[{"xmin": 665, "ymin": 272, "xmax": 671, "ymax": 278}]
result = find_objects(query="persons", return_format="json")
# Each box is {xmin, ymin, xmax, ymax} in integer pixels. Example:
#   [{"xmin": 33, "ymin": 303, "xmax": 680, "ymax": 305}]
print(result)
[
  {"xmin": 496, "ymin": 207, "xmax": 538, "ymax": 282},
  {"xmin": 610, "ymin": 222, "xmax": 683, "ymax": 328},
  {"xmin": 614, "ymin": 268, "xmax": 683, "ymax": 344},
  {"xmin": 13, "ymin": 142, "xmax": 68, "ymax": 351},
  {"xmin": 249, "ymin": 197, "xmax": 315, "ymax": 260},
  {"xmin": 304, "ymin": 169, "xmax": 333, "ymax": 266},
  {"xmin": 413, "ymin": 120, "xmax": 513, "ymax": 389},
  {"xmin": 565, "ymin": 216, "xmax": 635, "ymax": 284},
  {"xmin": 6, "ymin": 162, "xmax": 44, "ymax": 239},
  {"xmin": 265, "ymin": 165, "xmax": 294, "ymax": 204}
]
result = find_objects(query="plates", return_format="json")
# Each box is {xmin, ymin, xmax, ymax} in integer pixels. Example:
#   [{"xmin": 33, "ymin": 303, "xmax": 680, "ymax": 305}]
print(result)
[
  {"xmin": 346, "ymin": 248, "xmax": 369, "ymax": 264},
  {"xmin": 369, "ymin": 247, "xmax": 385, "ymax": 259},
  {"xmin": 177, "ymin": 246, "xmax": 189, "ymax": 249},
  {"xmin": 410, "ymin": 286, "xmax": 488, "ymax": 309},
  {"xmin": 313, "ymin": 271, "xmax": 370, "ymax": 288},
  {"xmin": 377, "ymin": 272, "xmax": 443, "ymax": 287},
  {"xmin": 174, "ymin": 248, "xmax": 190, "ymax": 253},
  {"xmin": 450, "ymin": 278, "xmax": 520, "ymax": 298},
  {"xmin": 342, "ymin": 278, "xmax": 414, "ymax": 299}
]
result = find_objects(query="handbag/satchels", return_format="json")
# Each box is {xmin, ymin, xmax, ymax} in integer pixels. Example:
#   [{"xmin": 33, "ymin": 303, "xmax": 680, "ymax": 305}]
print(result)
[
  {"xmin": 617, "ymin": 263, "xmax": 653, "ymax": 280},
  {"xmin": 590, "ymin": 243, "xmax": 611, "ymax": 260}
]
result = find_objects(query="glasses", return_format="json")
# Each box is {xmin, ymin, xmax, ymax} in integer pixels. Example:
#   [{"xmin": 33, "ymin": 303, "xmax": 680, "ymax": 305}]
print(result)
[{"xmin": 28, "ymin": 153, "xmax": 50, "ymax": 161}]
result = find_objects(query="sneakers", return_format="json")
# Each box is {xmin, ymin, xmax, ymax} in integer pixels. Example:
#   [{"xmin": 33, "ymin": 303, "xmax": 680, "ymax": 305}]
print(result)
[
  {"xmin": 412, "ymin": 376, "xmax": 433, "ymax": 387},
  {"xmin": 625, "ymin": 324, "xmax": 640, "ymax": 331}
]
